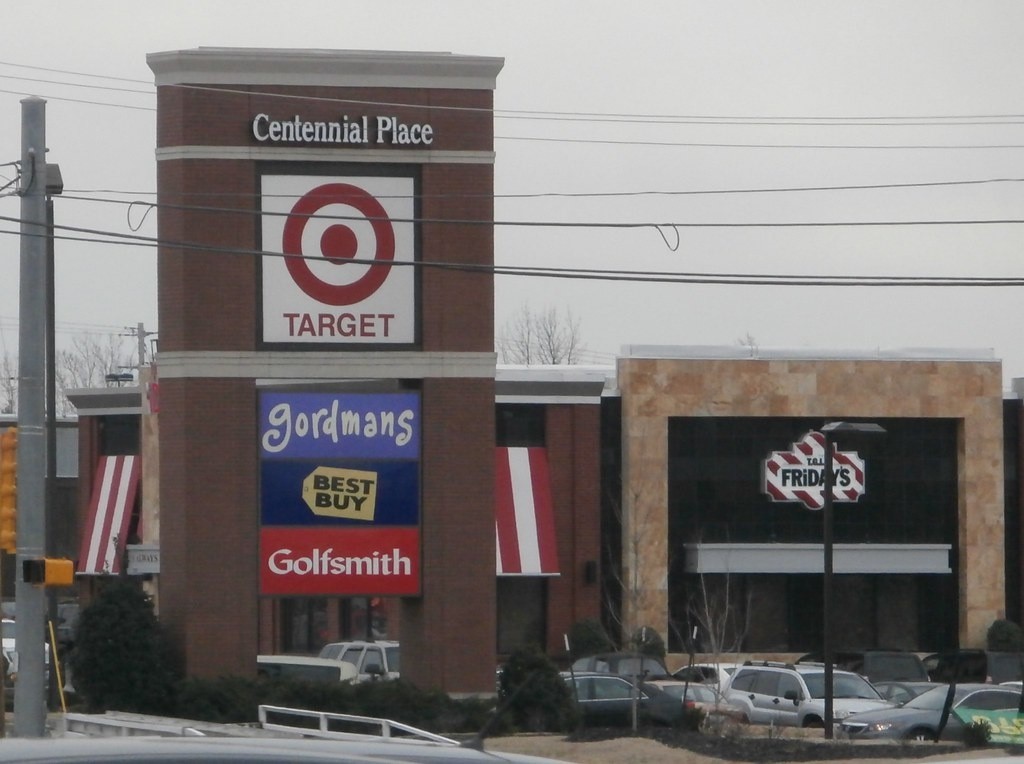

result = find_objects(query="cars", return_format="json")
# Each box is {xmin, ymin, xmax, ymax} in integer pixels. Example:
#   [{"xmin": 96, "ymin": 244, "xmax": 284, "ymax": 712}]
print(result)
[
  {"xmin": 257, "ymin": 640, "xmax": 400, "ymax": 685},
  {"xmin": 558, "ymin": 650, "xmax": 1023, "ymax": 742},
  {"xmin": 2, "ymin": 618, "xmax": 50, "ymax": 687}
]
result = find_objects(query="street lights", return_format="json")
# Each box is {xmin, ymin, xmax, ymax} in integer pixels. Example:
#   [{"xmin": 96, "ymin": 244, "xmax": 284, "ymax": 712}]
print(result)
[{"xmin": 819, "ymin": 421, "xmax": 887, "ymax": 739}]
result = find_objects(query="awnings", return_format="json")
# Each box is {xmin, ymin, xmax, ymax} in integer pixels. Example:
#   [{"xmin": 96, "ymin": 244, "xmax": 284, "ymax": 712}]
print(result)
[
  {"xmin": 496, "ymin": 446, "xmax": 561, "ymax": 577},
  {"xmin": 75, "ymin": 455, "xmax": 140, "ymax": 576}
]
